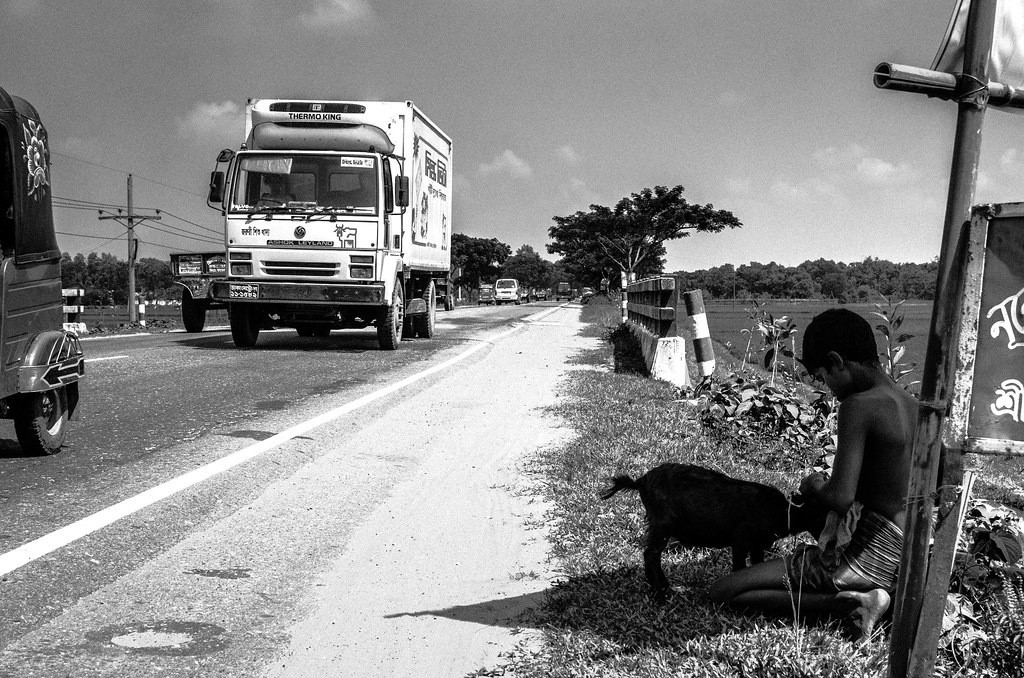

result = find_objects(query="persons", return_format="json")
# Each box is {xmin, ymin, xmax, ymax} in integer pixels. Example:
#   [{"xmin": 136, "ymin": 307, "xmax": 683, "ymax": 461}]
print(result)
[
  {"xmin": 349, "ymin": 170, "xmax": 376, "ymax": 207},
  {"xmin": 600, "ymin": 278, "xmax": 610, "ymax": 295},
  {"xmin": 260, "ymin": 173, "xmax": 297, "ymax": 207},
  {"xmin": 710, "ymin": 307, "xmax": 920, "ymax": 653}
]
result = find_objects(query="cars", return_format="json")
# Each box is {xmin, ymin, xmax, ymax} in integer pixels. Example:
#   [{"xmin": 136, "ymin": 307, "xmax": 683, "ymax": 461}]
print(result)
[
  {"xmin": 477, "ymin": 283, "xmax": 495, "ymax": 304},
  {"xmin": 0, "ymin": 87, "xmax": 87, "ymax": 455},
  {"xmin": 520, "ymin": 285, "xmax": 553, "ymax": 303}
]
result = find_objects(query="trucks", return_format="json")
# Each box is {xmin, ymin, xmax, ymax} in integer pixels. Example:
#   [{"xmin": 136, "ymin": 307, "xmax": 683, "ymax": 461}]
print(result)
[
  {"xmin": 207, "ymin": 96, "xmax": 453, "ymax": 350},
  {"xmin": 169, "ymin": 252, "xmax": 228, "ymax": 334}
]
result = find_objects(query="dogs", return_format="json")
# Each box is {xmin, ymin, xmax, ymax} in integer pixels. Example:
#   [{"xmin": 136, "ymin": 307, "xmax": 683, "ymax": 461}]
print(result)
[{"xmin": 596, "ymin": 457, "xmax": 831, "ymax": 604}]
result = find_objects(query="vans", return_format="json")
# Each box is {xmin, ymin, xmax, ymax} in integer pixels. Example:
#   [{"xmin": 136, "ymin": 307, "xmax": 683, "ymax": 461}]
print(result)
[
  {"xmin": 556, "ymin": 282, "xmax": 572, "ymax": 301},
  {"xmin": 494, "ymin": 279, "xmax": 522, "ymax": 305},
  {"xmin": 581, "ymin": 287, "xmax": 591, "ymax": 297}
]
